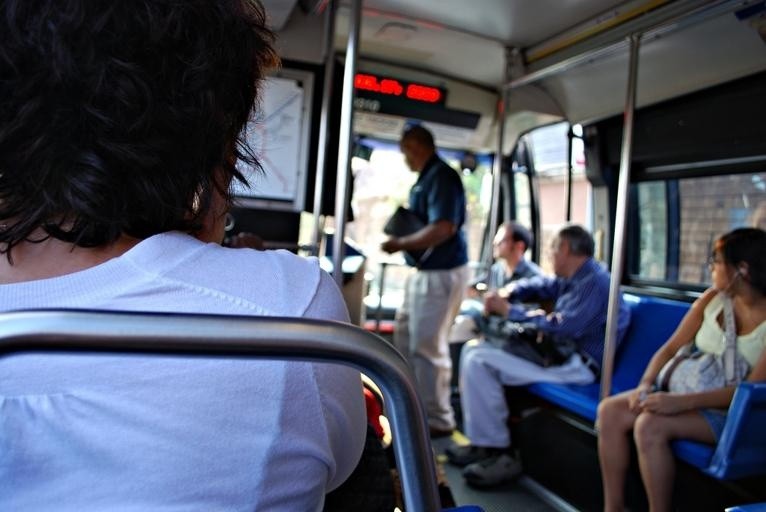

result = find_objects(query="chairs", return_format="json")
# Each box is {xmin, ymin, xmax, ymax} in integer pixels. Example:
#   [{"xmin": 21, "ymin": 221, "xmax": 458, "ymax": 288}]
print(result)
[{"xmin": 524, "ymin": 285, "xmax": 766, "ymax": 482}]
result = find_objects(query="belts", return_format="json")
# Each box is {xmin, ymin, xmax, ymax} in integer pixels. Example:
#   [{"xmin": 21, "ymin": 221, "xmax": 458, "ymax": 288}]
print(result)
[{"xmin": 576, "ymin": 350, "xmax": 601, "ymax": 378}]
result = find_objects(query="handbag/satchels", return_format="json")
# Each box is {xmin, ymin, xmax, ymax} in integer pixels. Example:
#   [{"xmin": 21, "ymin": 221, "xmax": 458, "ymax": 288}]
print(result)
[
  {"xmin": 656, "ymin": 298, "xmax": 748, "ymax": 393},
  {"xmin": 384, "ymin": 206, "xmax": 436, "ymax": 266},
  {"xmin": 472, "ymin": 310, "xmax": 576, "ymax": 368}
]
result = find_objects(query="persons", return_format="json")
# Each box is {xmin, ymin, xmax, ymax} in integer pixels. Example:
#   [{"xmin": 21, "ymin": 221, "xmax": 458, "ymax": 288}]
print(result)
[
  {"xmin": 381, "ymin": 126, "xmax": 467, "ymax": 441},
  {"xmin": 594, "ymin": 229, "xmax": 766, "ymax": 512},
  {"xmin": 0, "ymin": 0, "xmax": 368, "ymax": 511}
]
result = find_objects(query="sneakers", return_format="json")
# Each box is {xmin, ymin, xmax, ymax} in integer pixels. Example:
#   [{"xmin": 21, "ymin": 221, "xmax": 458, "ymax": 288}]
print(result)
[{"xmin": 443, "ymin": 446, "xmax": 522, "ymax": 490}]
